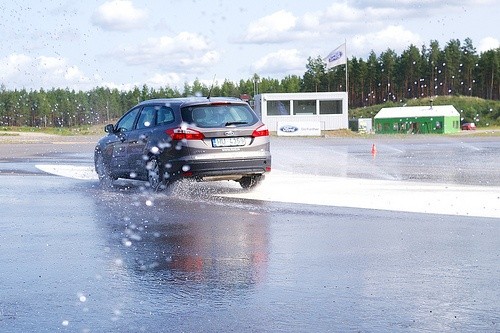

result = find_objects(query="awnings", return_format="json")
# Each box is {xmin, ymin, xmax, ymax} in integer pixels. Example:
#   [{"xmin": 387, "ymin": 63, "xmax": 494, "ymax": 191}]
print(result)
[{"xmin": 374, "ymin": 104, "xmax": 460, "ymax": 134}]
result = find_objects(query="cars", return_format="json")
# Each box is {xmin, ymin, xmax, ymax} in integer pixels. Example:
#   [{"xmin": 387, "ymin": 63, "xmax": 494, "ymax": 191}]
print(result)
[{"xmin": 93, "ymin": 96, "xmax": 272, "ymax": 193}]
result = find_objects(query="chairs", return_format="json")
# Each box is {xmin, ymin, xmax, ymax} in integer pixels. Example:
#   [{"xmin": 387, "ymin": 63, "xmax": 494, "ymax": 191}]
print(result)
[
  {"xmin": 193, "ymin": 109, "xmax": 208, "ymax": 125},
  {"xmin": 211, "ymin": 111, "xmax": 225, "ymax": 124}
]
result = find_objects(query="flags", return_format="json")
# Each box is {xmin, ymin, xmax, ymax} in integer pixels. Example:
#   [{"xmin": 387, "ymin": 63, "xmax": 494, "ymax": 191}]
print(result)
[{"xmin": 323, "ymin": 43, "xmax": 346, "ymax": 69}]
